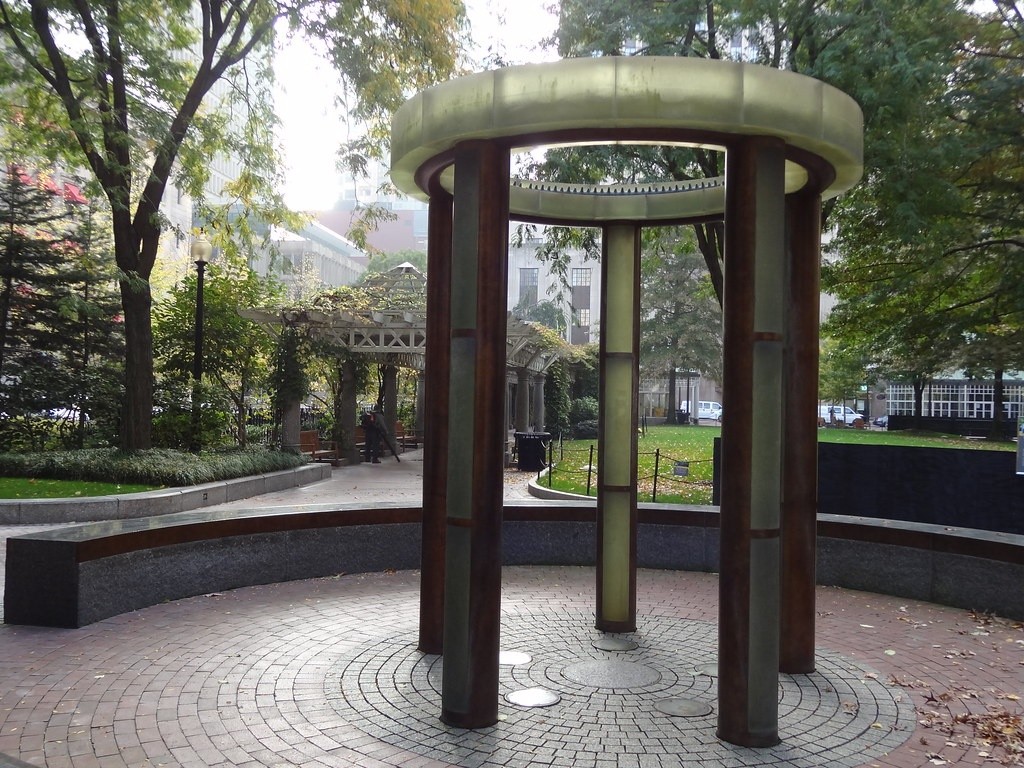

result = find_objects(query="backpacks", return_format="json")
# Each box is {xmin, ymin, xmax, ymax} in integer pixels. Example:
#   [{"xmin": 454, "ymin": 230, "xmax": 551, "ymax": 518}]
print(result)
[{"xmin": 358, "ymin": 411, "xmax": 382, "ymax": 432}]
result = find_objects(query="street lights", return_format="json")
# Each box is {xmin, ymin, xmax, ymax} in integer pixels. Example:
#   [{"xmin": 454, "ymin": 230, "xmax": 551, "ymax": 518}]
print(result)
[{"xmin": 191, "ymin": 226, "xmax": 212, "ymax": 454}]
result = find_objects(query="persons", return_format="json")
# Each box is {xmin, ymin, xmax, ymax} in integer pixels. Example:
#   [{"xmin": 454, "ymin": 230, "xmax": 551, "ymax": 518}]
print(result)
[
  {"xmin": 365, "ymin": 405, "xmax": 388, "ymax": 463},
  {"xmin": 830, "ymin": 407, "xmax": 835, "ymax": 423}
]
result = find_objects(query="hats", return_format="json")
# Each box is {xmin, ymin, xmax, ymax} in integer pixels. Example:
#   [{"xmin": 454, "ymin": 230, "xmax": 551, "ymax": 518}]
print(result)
[{"xmin": 374, "ymin": 404, "xmax": 383, "ymax": 410}]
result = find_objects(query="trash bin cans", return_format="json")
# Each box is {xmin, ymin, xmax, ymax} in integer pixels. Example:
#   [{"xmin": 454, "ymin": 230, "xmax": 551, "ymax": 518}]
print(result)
[
  {"xmin": 512, "ymin": 432, "xmax": 551, "ymax": 472},
  {"xmin": 677, "ymin": 413, "xmax": 690, "ymax": 423}
]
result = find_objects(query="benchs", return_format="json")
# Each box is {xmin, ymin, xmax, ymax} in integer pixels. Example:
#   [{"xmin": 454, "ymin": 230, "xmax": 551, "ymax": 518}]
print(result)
[
  {"xmin": 355, "ymin": 426, "xmax": 384, "ymax": 456},
  {"xmin": 396, "ymin": 422, "xmax": 420, "ymax": 452},
  {"xmin": 852, "ymin": 418, "xmax": 871, "ymax": 430},
  {"xmin": 302, "ymin": 430, "xmax": 339, "ymax": 466}
]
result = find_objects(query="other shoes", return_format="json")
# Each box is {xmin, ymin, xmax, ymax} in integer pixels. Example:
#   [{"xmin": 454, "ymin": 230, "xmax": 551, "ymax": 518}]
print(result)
[
  {"xmin": 365, "ymin": 458, "xmax": 372, "ymax": 462},
  {"xmin": 372, "ymin": 459, "xmax": 381, "ymax": 464}
]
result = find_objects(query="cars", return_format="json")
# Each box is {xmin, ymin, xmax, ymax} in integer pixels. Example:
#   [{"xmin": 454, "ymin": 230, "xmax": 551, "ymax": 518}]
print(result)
[{"xmin": 873, "ymin": 415, "xmax": 888, "ymax": 428}]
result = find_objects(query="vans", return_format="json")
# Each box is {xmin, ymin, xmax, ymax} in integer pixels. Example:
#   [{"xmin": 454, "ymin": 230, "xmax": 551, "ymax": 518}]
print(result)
[
  {"xmin": 680, "ymin": 400, "xmax": 722, "ymax": 422},
  {"xmin": 818, "ymin": 406, "xmax": 866, "ymax": 426}
]
